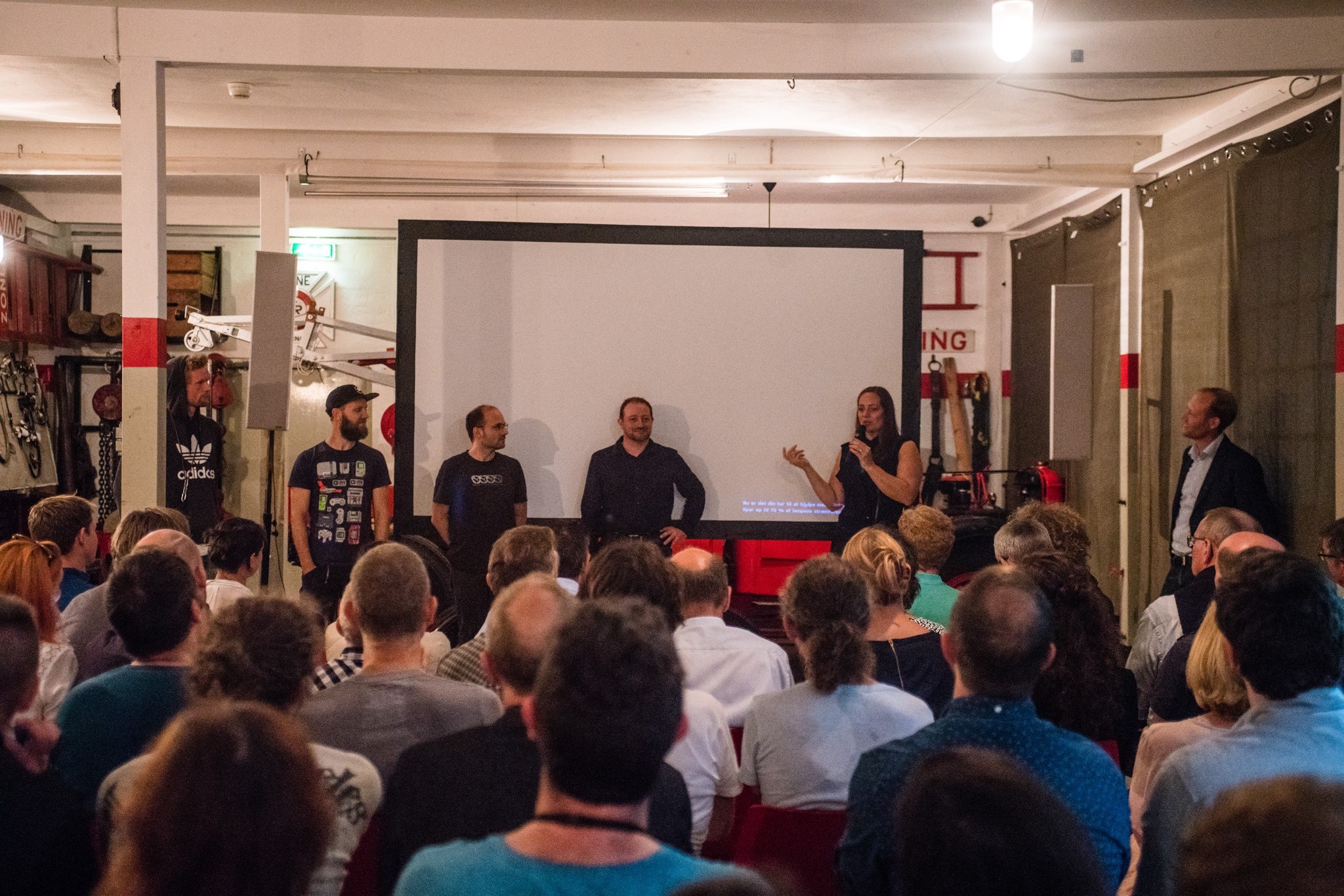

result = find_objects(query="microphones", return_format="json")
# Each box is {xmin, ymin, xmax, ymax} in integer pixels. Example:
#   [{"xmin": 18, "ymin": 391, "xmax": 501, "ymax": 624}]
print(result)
[{"xmin": 845, "ymin": 425, "xmax": 866, "ymax": 462}]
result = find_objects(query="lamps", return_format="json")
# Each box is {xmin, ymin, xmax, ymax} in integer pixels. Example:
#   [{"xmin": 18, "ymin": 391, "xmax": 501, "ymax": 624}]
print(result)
[{"xmin": 285, "ymin": 151, "xmax": 729, "ymax": 197}]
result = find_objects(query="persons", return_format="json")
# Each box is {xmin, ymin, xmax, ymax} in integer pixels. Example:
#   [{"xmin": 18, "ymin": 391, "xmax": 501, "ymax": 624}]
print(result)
[
  {"xmin": 166, "ymin": 353, "xmax": 225, "ymax": 544},
  {"xmin": 993, "ymin": 502, "xmax": 1125, "ymax": 738},
  {"xmin": 740, "ymin": 552, "xmax": 935, "ymax": 810},
  {"xmin": 97, "ymin": 697, "xmax": 332, "ymax": 896},
  {"xmin": 0, "ymin": 495, "xmax": 796, "ymax": 855},
  {"xmin": 431, "ymin": 405, "xmax": 528, "ymax": 645},
  {"xmin": 898, "ymin": 505, "xmax": 966, "ymax": 634},
  {"xmin": 0, "ymin": 593, "xmax": 80, "ymax": 896},
  {"xmin": 341, "ymin": 571, "xmax": 694, "ymax": 896},
  {"xmin": 782, "ymin": 386, "xmax": 924, "ymax": 558},
  {"xmin": 1159, "ymin": 388, "xmax": 1275, "ymax": 595},
  {"xmin": 833, "ymin": 563, "xmax": 1134, "ymax": 896},
  {"xmin": 288, "ymin": 384, "xmax": 392, "ymax": 629},
  {"xmin": 1126, "ymin": 507, "xmax": 1344, "ymax": 896},
  {"xmin": 840, "ymin": 526, "xmax": 956, "ymax": 723},
  {"xmin": 394, "ymin": 596, "xmax": 730, "ymax": 896},
  {"xmin": 95, "ymin": 593, "xmax": 383, "ymax": 896},
  {"xmin": 580, "ymin": 397, "xmax": 705, "ymax": 558}
]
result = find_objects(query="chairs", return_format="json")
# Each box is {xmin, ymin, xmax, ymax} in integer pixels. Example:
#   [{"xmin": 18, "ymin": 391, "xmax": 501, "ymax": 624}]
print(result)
[{"xmin": 696, "ymin": 725, "xmax": 846, "ymax": 896}]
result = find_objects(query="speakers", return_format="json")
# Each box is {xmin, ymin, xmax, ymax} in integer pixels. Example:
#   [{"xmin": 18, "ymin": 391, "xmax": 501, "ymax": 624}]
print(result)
[
  {"xmin": 245, "ymin": 251, "xmax": 299, "ymax": 432},
  {"xmin": 1049, "ymin": 284, "xmax": 1093, "ymax": 458}
]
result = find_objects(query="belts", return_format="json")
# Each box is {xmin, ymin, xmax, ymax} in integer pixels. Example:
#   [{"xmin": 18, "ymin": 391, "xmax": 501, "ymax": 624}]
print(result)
[{"xmin": 1171, "ymin": 555, "xmax": 1192, "ymax": 567}]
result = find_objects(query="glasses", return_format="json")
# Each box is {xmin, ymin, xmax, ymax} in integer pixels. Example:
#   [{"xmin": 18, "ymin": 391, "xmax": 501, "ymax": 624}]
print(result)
[
  {"xmin": 483, "ymin": 424, "xmax": 508, "ymax": 430},
  {"xmin": 1316, "ymin": 550, "xmax": 1335, "ymax": 562},
  {"xmin": 1187, "ymin": 536, "xmax": 1203, "ymax": 547}
]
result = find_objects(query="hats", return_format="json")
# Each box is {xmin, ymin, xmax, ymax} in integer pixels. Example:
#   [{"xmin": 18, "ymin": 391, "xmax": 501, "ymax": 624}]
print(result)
[{"xmin": 325, "ymin": 384, "xmax": 380, "ymax": 408}]
